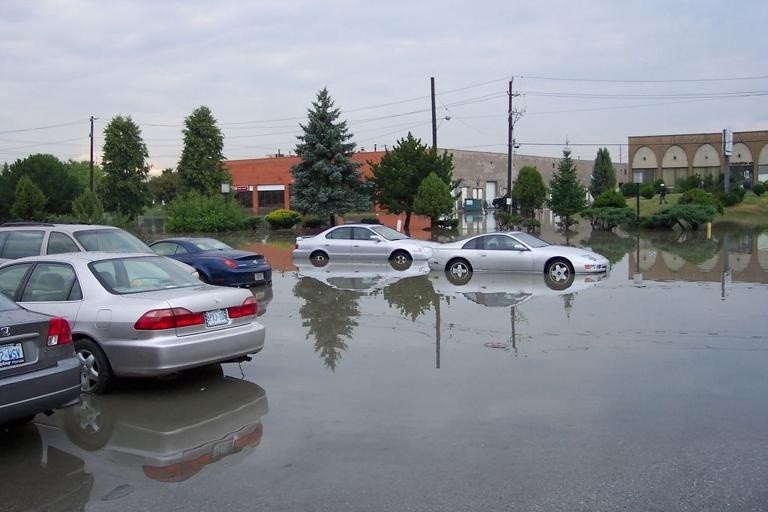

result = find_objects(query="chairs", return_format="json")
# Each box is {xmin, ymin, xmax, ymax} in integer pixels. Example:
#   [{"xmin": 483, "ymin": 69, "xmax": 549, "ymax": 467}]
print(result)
[{"xmin": 34, "ymin": 272, "xmax": 64, "ymax": 300}]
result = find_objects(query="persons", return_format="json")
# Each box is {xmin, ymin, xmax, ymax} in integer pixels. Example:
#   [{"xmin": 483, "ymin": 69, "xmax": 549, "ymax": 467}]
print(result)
[
  {"xmin": 658, "ymin": 180, "xmax": 667, "ymax": 205},
  {"xmin": 698, "ymin": 181, "xmax": 705, "ymax": 190}
]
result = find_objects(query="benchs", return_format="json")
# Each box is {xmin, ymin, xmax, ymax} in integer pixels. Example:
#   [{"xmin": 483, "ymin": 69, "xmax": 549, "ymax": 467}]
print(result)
[{"xmin": 65, "ymin": 271, "xmax": 114, "ymax": 300}]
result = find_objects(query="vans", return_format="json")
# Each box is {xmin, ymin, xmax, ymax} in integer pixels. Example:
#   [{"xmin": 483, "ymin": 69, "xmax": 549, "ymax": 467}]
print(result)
[{"xmin": 0, "ymin": 220, "xmax": 200, "ymax": 283}]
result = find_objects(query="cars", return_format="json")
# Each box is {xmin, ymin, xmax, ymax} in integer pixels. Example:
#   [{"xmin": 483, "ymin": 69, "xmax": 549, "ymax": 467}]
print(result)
[
  {"xmin": 0, "ymin": 290, "xmax": 83, "ymax": 432},
  {"xmin": 289, "ymin": 223, "xmax": 433, "ymax": 272},
  {"xmin": 144, "ymin": 234, "xmax": 273, "ymax": 288},
  {"xmin": 427, "ymin": 228, "xmax": 615, "ymax": 293},
  {"xmin": 0, "ymin": 249, "xmax": 267, "ymax": 394}
]
